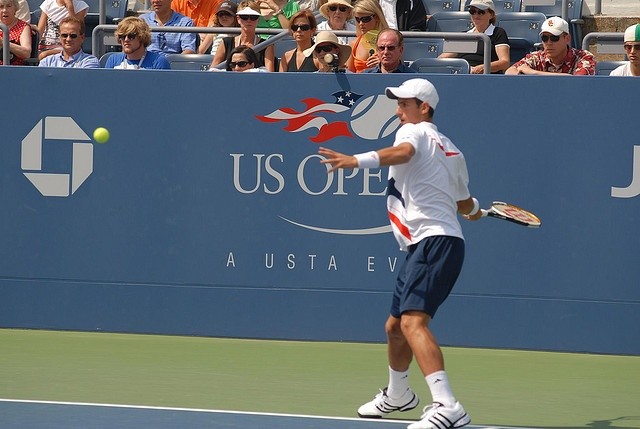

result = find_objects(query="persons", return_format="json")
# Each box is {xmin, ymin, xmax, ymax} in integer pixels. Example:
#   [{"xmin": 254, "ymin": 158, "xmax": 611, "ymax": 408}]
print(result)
[
  {"xmin": 610, "ymin": 23, "xmax": 640, "ymax": 77},
  {"xmin": 138, "ymin": 1, "xmax": 196, "ymax": 54},
  {"xmin": 210, "ymin": 46, "xmax": 268, "ymax": 72},
  {"xmin": 299, "ymin": 1, "xmax": 326, "ymax": 23},
  {"xmin": 0, "ymin": 0, "xmax": 32, "ymax": 66},
  {"xmin": 16, "ymin": 1, "xmax": 31, "ymax": 24},
  {"xmin": 303, "ymin": 31, "xmax": 354, "ymax": 74},
  {"xmin": 209, "ymin": 0, "xmax": 276, "ymax": 72},
  {"xmin": 376, "ymin": 1, "xmax": 426, "ymax": 31},
  {"xmin": 505, "ymin": 16, "xmax": 595, "ymax": 75},
  {"xmin": 362, "ymin": 27, "xmax": 420, "ymax": 73},
  {"xmin": 104, "ymin": 15, "xmax": 172, "ymax": 69},
  {"xmin": 315, "ymin": 1, "xmax": 359, "ymax": 45},
  {"xmin": 196, "ymin": 2, "xmax": 240, "ymax": 55},
  {"xmin": 172, "ymin": 0, "xmax": 222, "ymax": 39},
  {"xmin": 345, "ymin": 0, "xmax": 388, "ymax": 74},
  {"xmin": 278, "ymin": 8, "xmax": 319, "ymax": 73},
  {"xmin": 257, "ymin": 0, "xmax": 300, "ymax": 29},
  {"xmin": 34, "ymin": 0, "xmax": 87, "ymax": 56},
  {"xmin": 37, "ymin": 18, "xmax": 98, "ymax": 67},
  {"xmin": 317, "ymin": 79, "xmax": 483, "ymax": 429},
  {"xmin": 437, "ymin": 0, "xmax": 511, "ymax": 75}
]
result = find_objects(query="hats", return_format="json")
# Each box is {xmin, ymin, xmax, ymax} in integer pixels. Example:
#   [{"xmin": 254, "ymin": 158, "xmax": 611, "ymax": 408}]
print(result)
[
  {"xmin": 624, "ymin": 23, "xmax": 640, "ymax": 43},
  {"xmin": 319, "ymin": 0, "xmax": 354, "ymax": 19},
  {"xmin": 215, "ymin": 2, "xmax": 237, "ymax": 17},
  {"xmin": 539, "ymin": 16, "xmax": 569, "ymax": 35},
  {"xmin": 466, "ymin": 1, "xmax": 496, "ymax": 12},
  {"xmin": 384, "ymin": 78, "xmax": 440, "ymax": 110},
  {"xmin": 303, "ymin": 31, "xmax": 352, "ymax": 64},
  {"xmin": 236, "ymin": 7, "xmax": 262, "ymax": 16}
]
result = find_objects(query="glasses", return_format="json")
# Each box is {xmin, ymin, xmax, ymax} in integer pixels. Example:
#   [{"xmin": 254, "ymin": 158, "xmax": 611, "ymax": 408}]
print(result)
[
  {"xmin": 330, "ymin": 6, "xmax": 348, "ymax": 12},
  {"xmin": 229, "ymin": 61, "xmax": 250, "ymax": 68},
  {"xmin": 377, "ymin": 45, "xmax": 398, "ymax": 51},
  {"xmin": 59, "ymin": 33, "xmax": 82, "ymax": 39},
  {"xmin": 118, "ymin": 31, "xmax": 138, "ymax": 41},
  {"xmin": 468, "ymin": 9, "xmax": 489, "ymax": 16},
  {"xmin": 291, "ymin": 24, "xmax": 312, "ymax": 31},
  {"xmin": 540, "ymin": 35, "xmax": 562, "ymax": 42},
  {"xmin": 315, "ymin": 45, "xmax": 334, "ymax": 53},
  {"xmin": 238, "ymin": 15, "xmax": 260, "ymax": 21},
  {"xmin": 623, "ymin": 45, "xmax": 640, "ymax": 51},
  {"xmin": 354, "ymin": 15, "xmax": 374, "ymax": 23}
]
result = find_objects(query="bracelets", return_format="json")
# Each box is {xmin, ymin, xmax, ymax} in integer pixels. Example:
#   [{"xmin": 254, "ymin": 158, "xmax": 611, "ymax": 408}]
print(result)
[
  {"xmin": 354, "ymin": 151, "xmax": 381, "ymax": 173},
  {"xmin": 467, "ymin": 198, "xmax": 480, "ymax": 217}
]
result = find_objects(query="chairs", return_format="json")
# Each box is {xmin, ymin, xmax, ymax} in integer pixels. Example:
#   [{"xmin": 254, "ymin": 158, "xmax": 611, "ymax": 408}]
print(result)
[
  {"xmin": 594, "ymin": 61, "xmax": 626, "ymax": 77},
  {"xmin": 26, "ymin": 1, "xmax": 45, "ymax": 25},
  {"xmin": 165, "ymin": 54, "xmax": 214, "ymax": 70},
  {"xmin": 494, "ymin": 11, "xmax": 547, "ymax": 59},
  {"xmin": 99, "ymin": 52, "xmax": 119, "ymax": 68},
  {"xmin": 524, "ymin": 0, "xmax": 583, "ymax": 47},
  {"xmin": 428, "ymin": 11, "xmax": 472, "ymax": 32},
  {"xmin": 464, "ymin": 0, "xmax": 522, "ymax": 13},
  {"xmin": 410, "ymin": 59, "xmax": 470, "ymax": 74},
  {"xmin": 23, "ymin": 32, "xmax": 39, "ymax": 66},
  {"xmin": 84, "ymin": 1, "xmax": 127, "ymax": 33},
  {"xmin": 423, "ymin": 0, "xmax": 461, "ymax": 13},
  {"xmin": 267, "ymin": 33, "xmax": 297, "ymax": 58},
  {"xmin": 401, "ymin": 37, "xmax": 445, "ymax": 60}
]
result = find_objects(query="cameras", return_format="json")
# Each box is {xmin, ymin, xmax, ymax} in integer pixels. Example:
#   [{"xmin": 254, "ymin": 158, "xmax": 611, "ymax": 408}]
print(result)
[{"xmin": 323, "ymin": 53, "xmax": 347, "ymax": 74}]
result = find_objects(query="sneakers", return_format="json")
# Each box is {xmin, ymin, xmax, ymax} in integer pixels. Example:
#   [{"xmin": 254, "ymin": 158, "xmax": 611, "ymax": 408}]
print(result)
[
  {"xmin": 407, "ymin": 401, "xmax": 471, "ymax": 429},
  {"xmin": 357, "ymin": 386, "xmax": 419, "ymax": 418}
]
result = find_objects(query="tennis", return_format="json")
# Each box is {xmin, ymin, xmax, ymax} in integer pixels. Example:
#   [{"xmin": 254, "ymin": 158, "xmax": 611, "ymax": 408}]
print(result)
[{"xmin": 91, "ymin": 126, "xmax": 113, "ymax": 144}]
